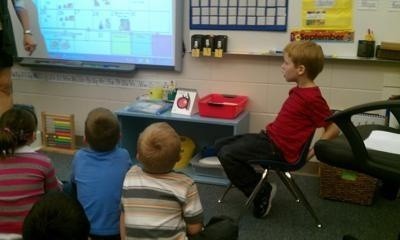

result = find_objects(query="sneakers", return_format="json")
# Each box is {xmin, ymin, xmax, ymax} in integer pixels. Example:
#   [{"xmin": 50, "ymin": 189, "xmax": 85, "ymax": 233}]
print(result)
[{"xmin": 252, "ymin": 181, "xmax": 277, "ymax": 218}]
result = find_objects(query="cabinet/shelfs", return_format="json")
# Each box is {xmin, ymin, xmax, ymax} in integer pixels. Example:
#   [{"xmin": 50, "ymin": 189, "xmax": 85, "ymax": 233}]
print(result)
[{"xmin": 116, "ymin": 104, "xmax": 251, "ymax": 187}]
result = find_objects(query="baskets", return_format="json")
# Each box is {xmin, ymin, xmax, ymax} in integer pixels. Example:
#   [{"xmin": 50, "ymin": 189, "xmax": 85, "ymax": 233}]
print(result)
[{"xmin": 318, "ymin": 162, "xmax": 378, "ymax": 206}]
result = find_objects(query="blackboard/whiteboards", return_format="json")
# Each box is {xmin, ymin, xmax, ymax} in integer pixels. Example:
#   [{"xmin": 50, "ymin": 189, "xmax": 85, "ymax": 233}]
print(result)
[{"xmin": 182, "ymin": 0, "xmax": 400, "ymax": 63}]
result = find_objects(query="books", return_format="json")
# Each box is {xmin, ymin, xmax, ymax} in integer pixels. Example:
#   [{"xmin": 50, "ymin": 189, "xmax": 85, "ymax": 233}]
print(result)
[{"xmin": 124, "ymin": 100, "xmax": 174, "ymax": 116}]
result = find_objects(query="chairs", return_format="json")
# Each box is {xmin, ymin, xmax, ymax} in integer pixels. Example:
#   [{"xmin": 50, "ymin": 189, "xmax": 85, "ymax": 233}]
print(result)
[{"xmin": 216, "ymin": 128, "xmax": 322, "ymax": 232}]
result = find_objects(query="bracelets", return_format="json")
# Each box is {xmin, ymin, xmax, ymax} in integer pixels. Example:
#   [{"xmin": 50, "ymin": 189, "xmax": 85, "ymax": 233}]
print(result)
[{"xmin": 23, "ymin": 30, "xmax": 34, "ymax": 35}]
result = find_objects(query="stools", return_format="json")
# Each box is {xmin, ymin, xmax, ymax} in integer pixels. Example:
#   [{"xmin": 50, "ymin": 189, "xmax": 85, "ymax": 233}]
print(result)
[{"xmin": 313, "ymin": 94, "xmax": 400, "ymax": 239}]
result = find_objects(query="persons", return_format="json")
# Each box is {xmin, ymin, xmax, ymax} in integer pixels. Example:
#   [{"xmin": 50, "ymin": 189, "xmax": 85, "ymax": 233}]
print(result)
[
  {"xmin": 214, "ymin": 41, "xmax": 340, "ymax": 219},
  {"xmin": 0, "ymin": 1, "xmax": 38, "ymax": 118},
  {"xmin": 0, "ymin": 103, "xmax": 239, "ymax": 240}
]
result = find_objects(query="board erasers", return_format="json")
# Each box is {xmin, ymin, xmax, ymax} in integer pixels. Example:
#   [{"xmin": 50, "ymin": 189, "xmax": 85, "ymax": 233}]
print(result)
[{"xmin": 376, "ymin": 44, "xmax": 400, "ymax": 61}]
